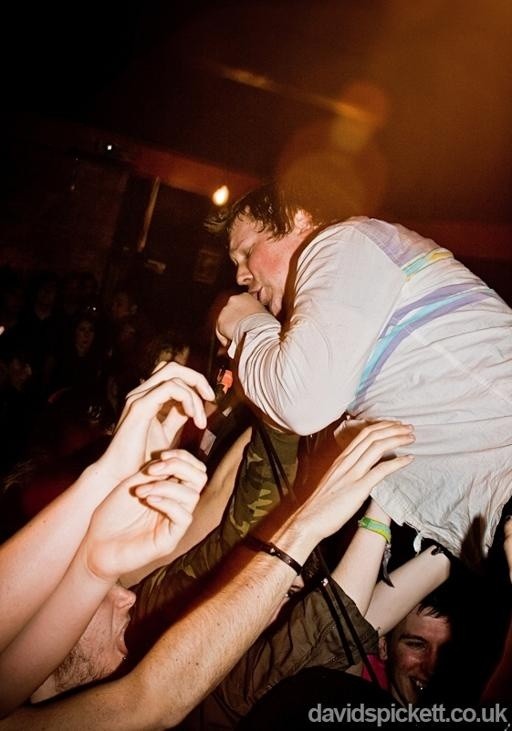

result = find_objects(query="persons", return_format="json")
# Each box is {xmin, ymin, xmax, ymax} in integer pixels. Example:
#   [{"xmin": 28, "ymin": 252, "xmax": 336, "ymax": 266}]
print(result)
[
  {"xmin": 0, "ymin": 224, "xmax": 511, "ymax": 731},
  {"xmin": 211, "ymin": 172, "xmax": 511, "ymax": 572}
]
